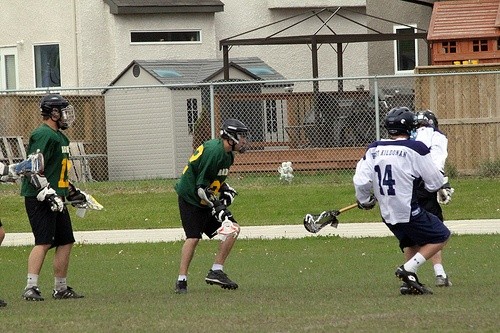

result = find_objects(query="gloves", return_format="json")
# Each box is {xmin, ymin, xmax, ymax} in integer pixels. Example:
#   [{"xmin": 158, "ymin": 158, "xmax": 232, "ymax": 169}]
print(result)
[
  {"xmin": 37, "ymin": 183, "xmax": 64, "ymax": 213},
  {"xmin": 219, "ymin": 186, "xmax": 239, "ymax": 207},
  {"xmin": 65, "ymin": 190, "xmax": 88, "ymax": 208},
  {"xmin": 356, "ymin": 196, "xmax": 376, "ymax": 210},
  {"xmin": 210, "ymin": 199, "xmax": 234, "ymax": 223},
  {"xmin": 6, "ymin": 162, "xmax": 25, "ymax": 179}
]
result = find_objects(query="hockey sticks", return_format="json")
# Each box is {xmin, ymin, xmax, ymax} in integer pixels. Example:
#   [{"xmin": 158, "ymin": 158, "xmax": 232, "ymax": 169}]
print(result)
[
  {"xmin": 216, "ymin": 199, "xmax": 239, "ymax": 242},
  {"xmin": 12, "ymin": 152, "xmax": 44, "ymax": 176},
  {"xmin": 302, "ymin": 202, "xmax": 360, "ymax": 234},
  {"xmin": 63, "ymin": 187, "xmax": 104, "ymax": 211}
]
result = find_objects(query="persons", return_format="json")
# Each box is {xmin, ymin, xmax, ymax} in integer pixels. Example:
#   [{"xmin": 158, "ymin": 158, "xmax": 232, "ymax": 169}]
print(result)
[
  {"xmin": 353, "ymin": 105, "xmax": 456, "ymax": 295},
  {"xmin": 0, "ymin": 152, "xmax": 45, "ymax": 307},
  {"xmin": 19, "ymin": 94, "xmax": 87, "ymax": 301},
  {"xmin": 172, "ymin": 118, "xmax": 249, "ymax": 291},
  {"xmin": 408, "ymin": 107, "xmax": 452, "ymax": 287}
]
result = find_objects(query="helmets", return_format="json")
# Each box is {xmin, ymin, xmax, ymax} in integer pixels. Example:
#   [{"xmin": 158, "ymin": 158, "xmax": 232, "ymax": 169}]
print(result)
[
  {"xmin": 40, "ymin": 94, "xmax": 75, "ymax": 130},
  {"xmin": 219, "ymin": 119, "xmax": 248, "ymax": 146},
  {"xmin": 383, "ymin": 107, "xmax": 418, "ymax": 135},
  {"xmin": 415, "ymin": 109, "xmax": 438, "ymax": 127}
]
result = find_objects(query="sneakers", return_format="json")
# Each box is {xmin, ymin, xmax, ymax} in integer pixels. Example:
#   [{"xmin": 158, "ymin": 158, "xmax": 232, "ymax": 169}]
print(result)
[
  {"xmin": 400, "ymin": 281, "xmax": 433, "ymax": 294},
  {"xmin": 51, "ymin": 286, "xmax": 84, "ymax": 299},
  {"xmin": 394, "ymin": 264, "xmax": 428, "ymax": 296},
  {"xmin": 434, "ymin": 274, "xmax": 452, "ymax": 287},
  {"xmin": 22, "ymin": 286, "xmax": 44, "ymax": 301},
  {"xmin": 204, "ymin": 269, "xmax": 239, "ymax": 290},
  {"xmin": 175, "ymin": 278, "xmax": 188, "ymax": 294}
]
